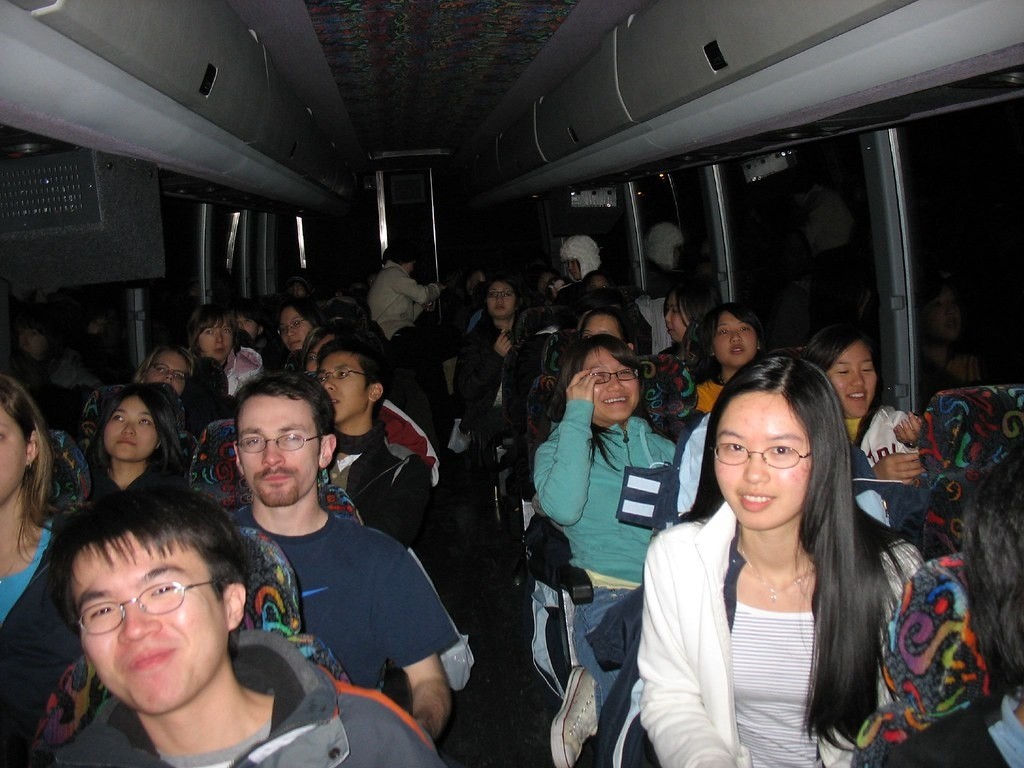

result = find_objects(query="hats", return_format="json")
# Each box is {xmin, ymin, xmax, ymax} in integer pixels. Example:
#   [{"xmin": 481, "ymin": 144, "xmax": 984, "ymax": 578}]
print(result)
[
  {"xmin": 286, "ymin": 268, "xmax": 312, "ymax": 293},
  {"xmin": 383, "ymin": 242, "xmax": 418, "ymax": 263}
]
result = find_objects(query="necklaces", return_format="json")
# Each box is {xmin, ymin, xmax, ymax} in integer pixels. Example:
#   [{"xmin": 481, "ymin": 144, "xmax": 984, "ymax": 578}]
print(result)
[{"xmin": 740, "ymin": 544, "xmax": 809, "ymax": 602}]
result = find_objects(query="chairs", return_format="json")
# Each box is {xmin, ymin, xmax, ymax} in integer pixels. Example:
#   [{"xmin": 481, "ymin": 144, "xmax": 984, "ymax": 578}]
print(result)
[
  {"xmin": 25, "ymin": 384, "xmax": 364, "ymax": 768},
  {"xmin": 849, "ymin": 383, "xmax": 1024, "ymax": 768},
  {"xmin": 503, "ymin": 306, "xmax": 700, "ymax": 481}
]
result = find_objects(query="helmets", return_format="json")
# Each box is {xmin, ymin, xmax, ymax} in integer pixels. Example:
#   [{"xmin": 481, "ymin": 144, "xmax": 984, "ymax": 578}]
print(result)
[
  {"xmin": 559, "ymin": 235, "xmax": 602, "ymax": 281},
  {"xmin": 648, "ymin": 223, "xmax": 684, "ymax": 270}
]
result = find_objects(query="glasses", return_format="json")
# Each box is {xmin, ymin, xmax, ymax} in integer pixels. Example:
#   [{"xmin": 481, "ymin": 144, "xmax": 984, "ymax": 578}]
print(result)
[
  {"xmin": 314, "ymin": 368, "xmax": 366, "ymax": 382},
  {"xmin": 711, "ymin": 442, "xmax": 812, "ymax": 469},
  {"xmin": 75, "ymin": 581, "xmax": 214, "ymax": 635},
  {"xmin": 589, "ymin": 369, "xmax": 638, "ymax": 383},
  {"xmin": 150, "ymin": 363, "xmax": 185, "ymax": 380},
  {"xmin": 486, "ymin": 289, "xmax": 513, "ymax": 298},
  {"xmin": 277, "ymin": 320, "xmax": 307, "ymax": 335},
  {"xmin": 307, "ymin": 352, "xmax": 319, "ymax": 363},
  {"xmin": 235, "ymin": 434, "xmax": 321, "ymax": 453}
]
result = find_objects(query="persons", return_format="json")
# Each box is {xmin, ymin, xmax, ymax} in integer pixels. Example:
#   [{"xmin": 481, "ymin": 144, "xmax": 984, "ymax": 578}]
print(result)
[
  {"xmin": 87, "ymin": 387, "xmax": 189, "ymax": 500},
  {"xmin": 636, "ymin": 355, "xmax": 923, "ymax": 768},
  {"xmin": 134, "ymin": 237, "xmax": 763, "ymax": 540},
  {"xmin": 888, "ymin": 448, "xmax": 1024, "ymax": 768},
  {"xmin": 231, "ymin": 373, "xmax": 459, "ymax": 741},
  {"xmin": 797, "ymin": 326, "xmax": 924, "ymax": 482},
  {"xmin": 532, "ymin": 333, "xmax": 677, "ymax": 768},
  {"xmin": 0, "ymin": 374, "xmax": 83, "ymax": 768},
  {"xmin": 45, "ymin": 484, "xmax": 448, "ymax": 768}
]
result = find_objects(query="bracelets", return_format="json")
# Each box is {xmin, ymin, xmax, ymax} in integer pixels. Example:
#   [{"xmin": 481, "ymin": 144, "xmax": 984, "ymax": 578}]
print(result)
[{"xmin": 904, "ymin": 443, "xmax": 919, "ymax": 447}]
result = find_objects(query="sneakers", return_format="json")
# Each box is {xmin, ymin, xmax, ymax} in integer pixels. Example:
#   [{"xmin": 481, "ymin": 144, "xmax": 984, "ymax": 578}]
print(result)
[{"xmin": 550, "ymin": 666, "xmax": 598, "ymax": 768}]
